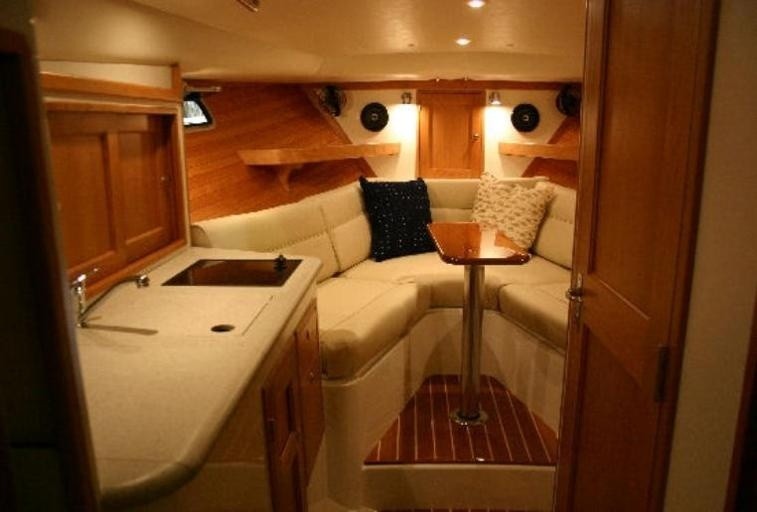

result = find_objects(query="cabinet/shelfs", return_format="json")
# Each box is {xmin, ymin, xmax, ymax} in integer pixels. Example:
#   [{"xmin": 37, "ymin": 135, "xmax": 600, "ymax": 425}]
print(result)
[
  {"xmin": 45, "ymin": 85, "xmax": 192, "ymax": 314},
  {"xmin": 261, "ymin": 298, "xmax": 325, "ymax": 512}
]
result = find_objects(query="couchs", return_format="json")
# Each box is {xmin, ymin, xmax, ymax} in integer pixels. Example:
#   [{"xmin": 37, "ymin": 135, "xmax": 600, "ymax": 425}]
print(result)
[{"xmin": 190, "ymin": 173, "xmax": 578, "ymax": 452}]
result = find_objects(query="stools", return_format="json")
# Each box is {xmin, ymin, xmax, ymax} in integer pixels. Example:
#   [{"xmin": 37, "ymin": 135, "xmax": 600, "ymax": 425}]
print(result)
[{"xmin": 427, "ymin": 223, "xmax": 533, "ymax": 420}]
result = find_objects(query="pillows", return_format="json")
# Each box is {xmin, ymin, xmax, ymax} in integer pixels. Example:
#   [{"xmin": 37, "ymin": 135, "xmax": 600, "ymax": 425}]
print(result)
[
  {"xmin": 472, "ymin": 173, "xmax": 558, "ymax": 251},
  {"xmin": 358, "ymin": 173, "xmax": 436, "ymax": 262}
]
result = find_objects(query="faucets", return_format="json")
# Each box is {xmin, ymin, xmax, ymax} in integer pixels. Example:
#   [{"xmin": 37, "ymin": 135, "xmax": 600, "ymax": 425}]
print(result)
[{"xmin": 69, "ymin": 272, "xmax": 150, "ymax": 323}]
